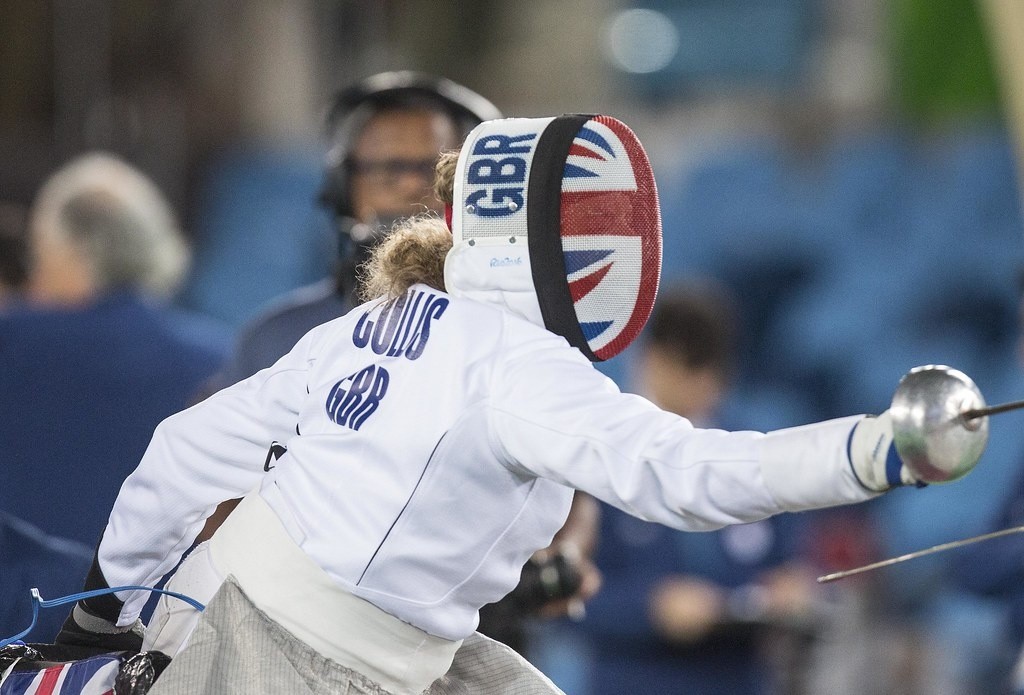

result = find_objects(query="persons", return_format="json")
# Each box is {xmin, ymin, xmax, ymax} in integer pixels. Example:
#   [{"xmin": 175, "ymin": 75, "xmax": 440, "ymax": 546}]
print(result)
[
  {"xmin": 51, "ymin": 66, "xmax": 950, "ymax": 695},
  {"xmin": 1, "ymin": 159, "xmax": 246, "ymax": 652}
]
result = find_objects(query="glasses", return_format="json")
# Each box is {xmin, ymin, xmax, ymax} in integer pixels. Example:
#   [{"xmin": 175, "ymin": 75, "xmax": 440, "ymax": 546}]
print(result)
[{"xmin": 343, "ymin": 156, "xmax": 443, "ymax": 191}]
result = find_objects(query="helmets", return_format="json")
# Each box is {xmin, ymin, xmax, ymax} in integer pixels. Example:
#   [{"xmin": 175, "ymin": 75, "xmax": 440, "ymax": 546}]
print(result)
[{"xmin": 443, "ymin": 114, "xmax": 662, "ymax": 360}]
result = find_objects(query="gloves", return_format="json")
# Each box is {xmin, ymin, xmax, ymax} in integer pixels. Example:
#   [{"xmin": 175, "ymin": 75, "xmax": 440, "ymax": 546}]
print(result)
[
  {"xmin": 52, "ymin": 593, "xmax": 147, "ymax": 660},
  {"xmin": 847, "ymin": 405, "xmax": 929, "ymax": 493}
]
